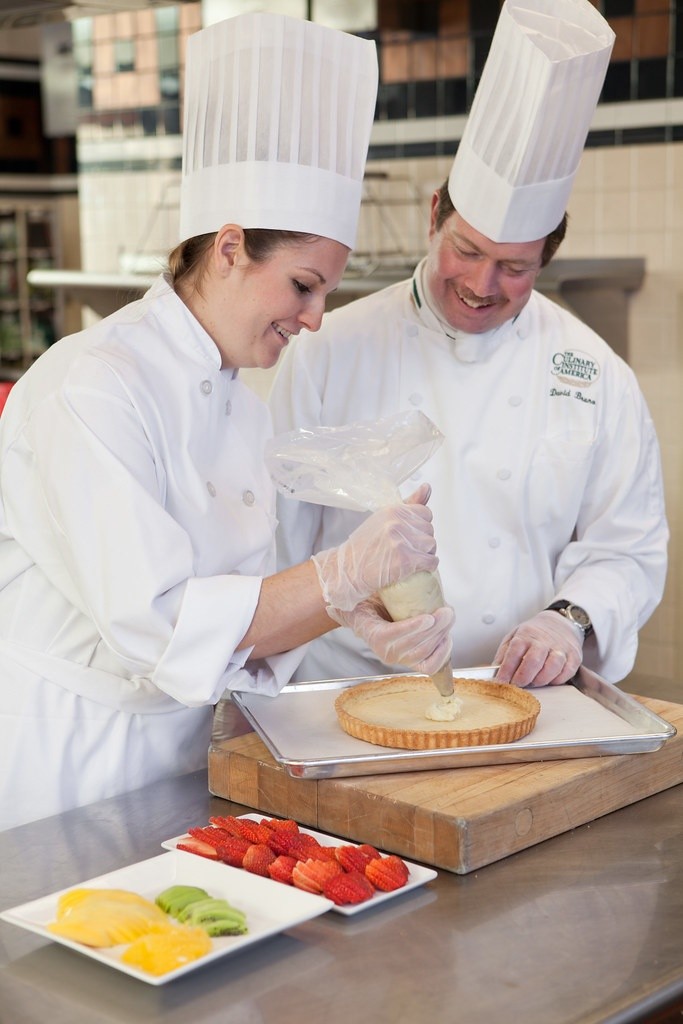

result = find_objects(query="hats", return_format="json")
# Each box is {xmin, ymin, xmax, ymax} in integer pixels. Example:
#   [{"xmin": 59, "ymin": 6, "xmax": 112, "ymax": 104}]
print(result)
[
  {"xmin": 445, "ymin": 1, "xmax": 620, "ymax": 246},
  {"xmin": 177, "ymin": 9, "xmax": 380, "ymax": 249}
]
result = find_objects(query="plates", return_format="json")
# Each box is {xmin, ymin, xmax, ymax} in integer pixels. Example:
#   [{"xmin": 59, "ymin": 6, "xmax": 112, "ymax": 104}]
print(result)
[
  {"xmin": 0, "ymin": 849, "xmax": 335, "ymax": 987},
  {"xmin": 160, "ymin": 812, "xmax": 438, "ymax": 917}
]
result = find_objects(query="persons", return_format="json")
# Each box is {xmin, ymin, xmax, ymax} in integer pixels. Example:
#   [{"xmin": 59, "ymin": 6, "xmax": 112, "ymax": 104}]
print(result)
[
  {"xmin": 0, "ymin": 155, "xmax": 453, "ymax": 836},
  {"xmin": 260, "ymin": 156, "xmax": 671, "ymax": 689}
]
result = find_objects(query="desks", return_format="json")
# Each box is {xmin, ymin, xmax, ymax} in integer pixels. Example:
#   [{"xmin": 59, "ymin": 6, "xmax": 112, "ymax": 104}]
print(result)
[{"xmin": 0, "ymin": 673, "xmax": 683, "ymax": 1024}]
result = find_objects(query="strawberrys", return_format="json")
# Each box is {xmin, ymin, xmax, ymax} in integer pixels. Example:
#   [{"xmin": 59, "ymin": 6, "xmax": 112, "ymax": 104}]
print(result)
[{"xmin": 177, "ymin": 815, "xmax": 408, "ymax": 904}]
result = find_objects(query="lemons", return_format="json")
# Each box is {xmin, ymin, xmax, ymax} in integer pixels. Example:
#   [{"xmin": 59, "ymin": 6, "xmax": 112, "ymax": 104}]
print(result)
[{"xmin": 48, "ymin": 888, "xmax": 211, "ymax": 972}]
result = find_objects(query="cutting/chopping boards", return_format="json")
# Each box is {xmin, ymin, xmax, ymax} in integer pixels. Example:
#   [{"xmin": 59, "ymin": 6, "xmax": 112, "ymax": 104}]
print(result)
[{"xmin": 208, "ymin": 692, "xmax": 683, "ymax": 874}]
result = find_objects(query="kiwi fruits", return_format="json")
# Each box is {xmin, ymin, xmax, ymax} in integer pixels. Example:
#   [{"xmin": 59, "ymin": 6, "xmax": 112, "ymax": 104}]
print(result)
[{"xmin": 156, "ymin": 887, "xmax": 247, "ymax": 936}]
